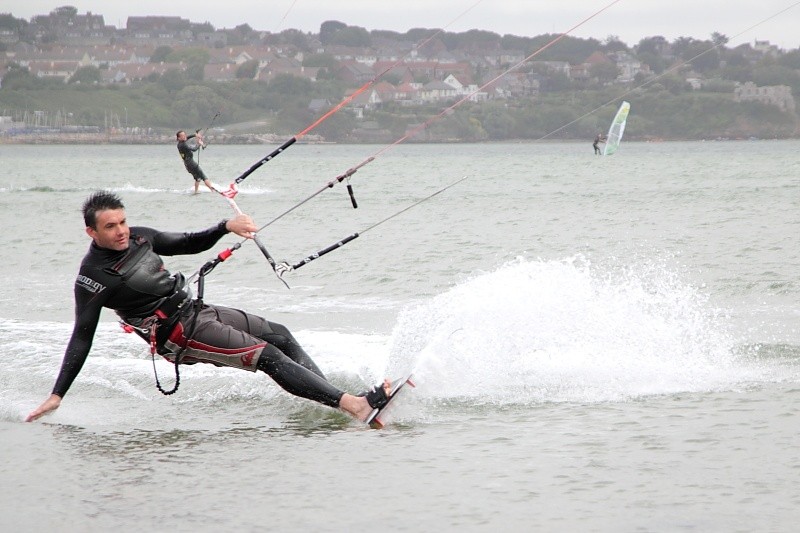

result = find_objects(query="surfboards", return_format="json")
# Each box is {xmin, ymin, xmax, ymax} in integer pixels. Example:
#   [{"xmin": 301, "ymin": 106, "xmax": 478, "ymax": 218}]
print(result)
[{"xmin": 365, "ymin": 373, "xmax": 415, "ymax": 429}]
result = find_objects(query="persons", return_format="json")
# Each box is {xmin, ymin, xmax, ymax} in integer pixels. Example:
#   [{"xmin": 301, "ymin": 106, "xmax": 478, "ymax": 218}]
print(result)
[
  {"xmin": 176, "ymin": 130, "xmax": 216, "ymax": 193},
  {"xmin": 593, "ymin": 133, "xmax": 607, "ymax": 155},
  {"xmin": 24, "ymin": 190, "xmax": 392, "ymax": 425}
]
